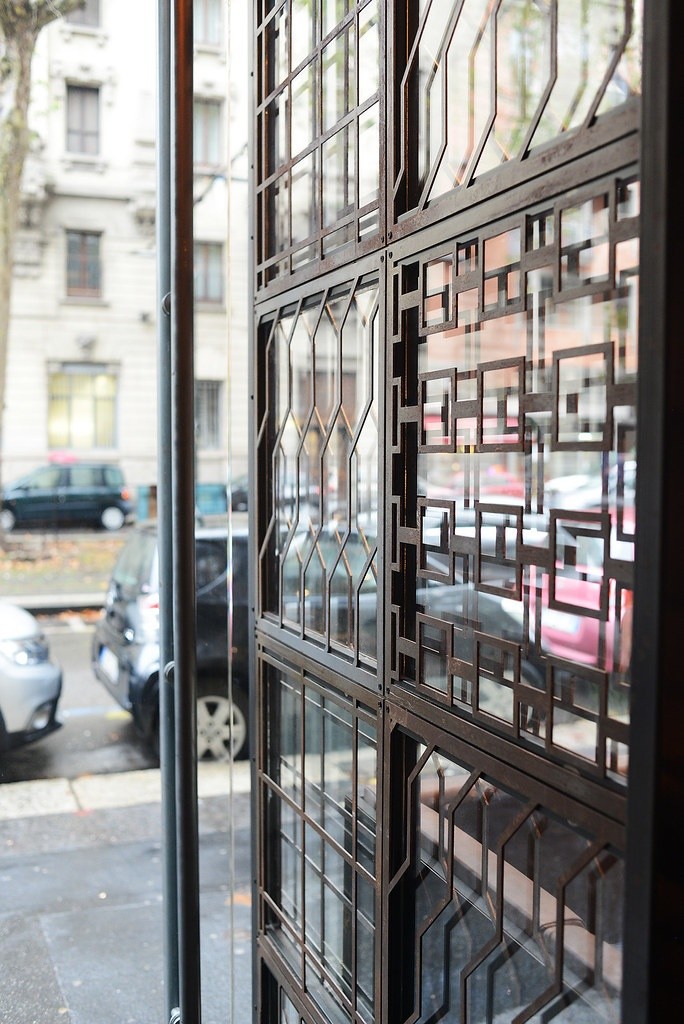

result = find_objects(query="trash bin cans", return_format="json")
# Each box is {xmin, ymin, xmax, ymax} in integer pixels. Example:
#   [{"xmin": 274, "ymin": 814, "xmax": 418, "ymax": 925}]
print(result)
[{"xmin": 136, "ymin": 482, "xmax": 228, "ymax": 520}]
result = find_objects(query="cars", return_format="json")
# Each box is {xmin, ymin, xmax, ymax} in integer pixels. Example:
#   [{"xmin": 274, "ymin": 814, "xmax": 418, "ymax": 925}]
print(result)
[
  {"xmin": 2, "ymin": 463, "xmax": 135, "ymax": 532},
  {"xmin": 0, "ymin": 602, "xmax": 63, "ymax": 757},
  {"xmin": 93, "ymin": 463, "xmax": 636, "ymax": 766}
]
